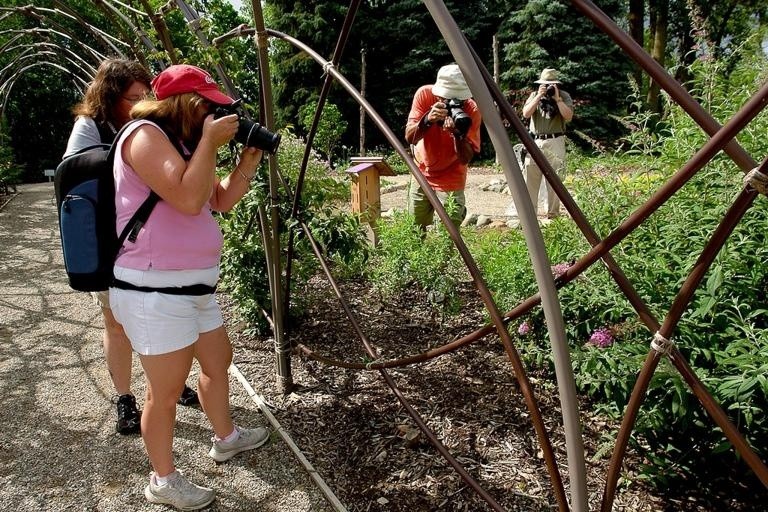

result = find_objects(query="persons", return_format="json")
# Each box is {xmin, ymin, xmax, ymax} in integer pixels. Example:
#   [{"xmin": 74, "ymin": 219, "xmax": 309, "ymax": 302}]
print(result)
[
  {"xmin": 521, "ymin": 67, "xmax": 577, "ymax": 226},
  {"xmin": 107, "ymin": 63, "xmax": 272, "ymax": 512},
  {"xmin": 61, "ymin": 56, "xmax": 200, "ymax": 435},
  {"xmin": 405, "ymin": 63, "xmax": 481, "ymax": 254}
]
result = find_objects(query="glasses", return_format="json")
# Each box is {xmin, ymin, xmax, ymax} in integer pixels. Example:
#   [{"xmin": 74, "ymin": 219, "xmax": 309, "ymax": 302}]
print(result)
[{"xmin": 123, "ymin": 94, "xmax": 139, "ymax": 107}]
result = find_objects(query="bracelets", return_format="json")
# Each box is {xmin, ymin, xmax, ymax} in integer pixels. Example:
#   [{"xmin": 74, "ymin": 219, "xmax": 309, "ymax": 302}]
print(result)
[
  {"xmin": 555, "ymin": 97, "xmax": 563, "ymax": 104},
  {"xmin": 237, "ymin": 165, "xmax": 251, "ymax": 183}
]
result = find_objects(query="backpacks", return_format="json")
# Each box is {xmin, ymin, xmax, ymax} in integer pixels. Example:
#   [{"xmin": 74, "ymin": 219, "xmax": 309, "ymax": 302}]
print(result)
[{"xmin": 55, "ymin": 121, "xmax": 159, "ymax": 292}]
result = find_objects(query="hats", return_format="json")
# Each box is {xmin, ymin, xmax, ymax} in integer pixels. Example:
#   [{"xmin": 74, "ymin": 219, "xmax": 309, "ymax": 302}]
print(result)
[
  {"xmin": 532, "ymin": 67, "xmax": 563, "ymax": 85},
  {"xmin": 431, "ymin": 66, "xmax": 474, "ymax": 101},
  {"xmin": 151, "ymin": 64, "xmax": 235, "ymax": 105}
]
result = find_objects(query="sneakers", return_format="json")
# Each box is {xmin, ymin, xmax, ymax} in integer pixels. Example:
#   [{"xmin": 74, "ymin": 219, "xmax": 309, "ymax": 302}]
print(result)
[
  {"xmin": 209, "ymin": 425, "xmax": 270, "ymax": 463},
  {"xmin": 145, "ymin": 471, "xmax": 216, "ymax": 512},
  {"xmin": 177, "ymin": 385, "xmax": 199, "ymax": 407},
  {"xmin": 111, "ymin": 395, "xmax": 140, "ymax": 433}
]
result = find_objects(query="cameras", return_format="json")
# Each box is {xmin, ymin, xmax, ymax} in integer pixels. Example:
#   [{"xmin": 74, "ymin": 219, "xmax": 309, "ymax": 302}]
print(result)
[
  {"xmin": 215, "ymin": 98, "xmax": 282, "ymax": 157},
  {"xmin": 437, "ymin": 95, "xmax": 473, "ymax": 139},
  {"xmin": 544, "ymin": 84, "xmax": 556, "ymax": 97}
]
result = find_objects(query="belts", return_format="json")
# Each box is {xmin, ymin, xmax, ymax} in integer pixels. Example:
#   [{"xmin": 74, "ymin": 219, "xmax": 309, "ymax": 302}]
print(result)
[{"xmin": 533, "ymin": 132, "xmax": 563, "ymax": 139}]
